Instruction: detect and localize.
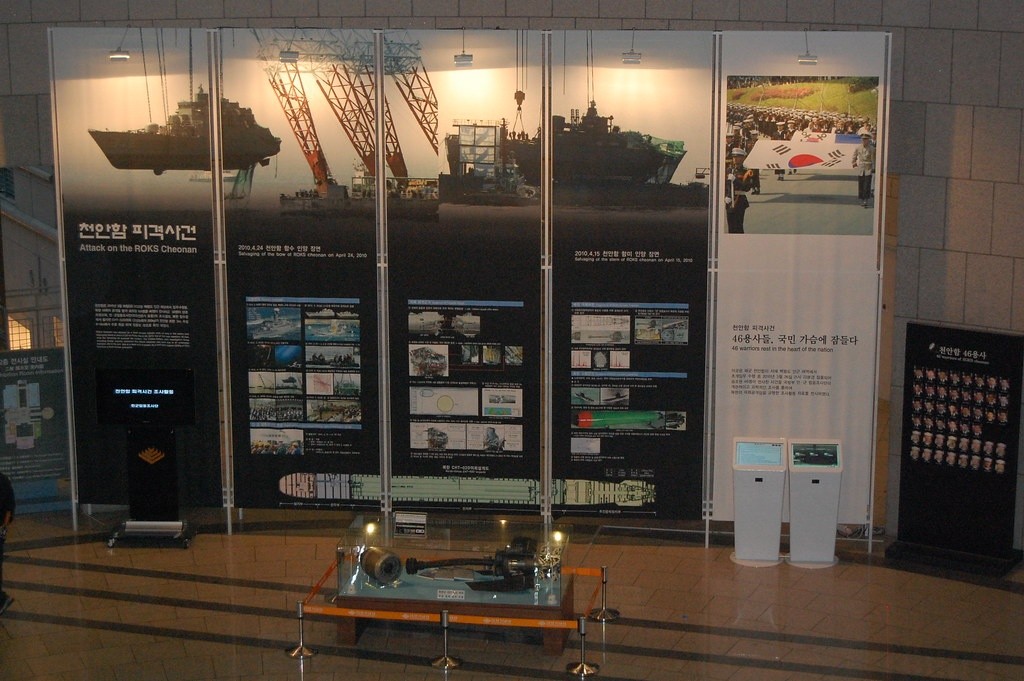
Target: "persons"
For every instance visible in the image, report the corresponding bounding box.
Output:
[724,99,876,236]
[310,351,355,368]
[251,439,304,456]
[315,403,362,424]
[908,365,1008,475]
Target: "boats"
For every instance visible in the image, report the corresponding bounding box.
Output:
[441,104,688,186]
[88,85,283,177]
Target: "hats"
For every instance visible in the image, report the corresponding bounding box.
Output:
[870,127,877,134]
[776,121,785,126]
[860,134,871,139]
[732,148,745,156]
[788,120,795,124]
[726,133,734,137]
[749,131,759,135]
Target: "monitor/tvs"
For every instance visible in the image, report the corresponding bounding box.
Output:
[735,442,781,466]
[791,444,839,467]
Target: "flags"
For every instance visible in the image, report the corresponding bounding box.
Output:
[740,138,865,174]
[789,130,864,147]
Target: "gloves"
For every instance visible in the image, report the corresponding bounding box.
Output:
[728,174,736,181]
[725,197,731,204]
[871,169,875,173]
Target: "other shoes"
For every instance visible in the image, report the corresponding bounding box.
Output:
[778,177,784,181]
[751,189,760,195]
